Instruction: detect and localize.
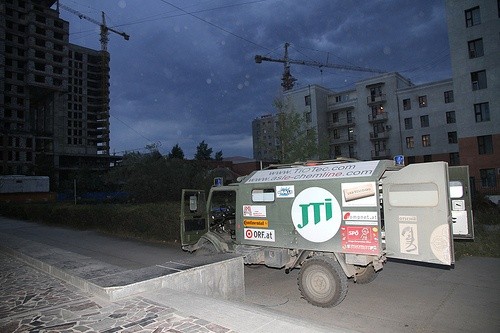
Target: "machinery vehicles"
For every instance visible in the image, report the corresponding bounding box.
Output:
[180,155,475,308]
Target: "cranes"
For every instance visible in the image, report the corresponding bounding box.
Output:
[56,2,130,51]
[254,41,387,90]
